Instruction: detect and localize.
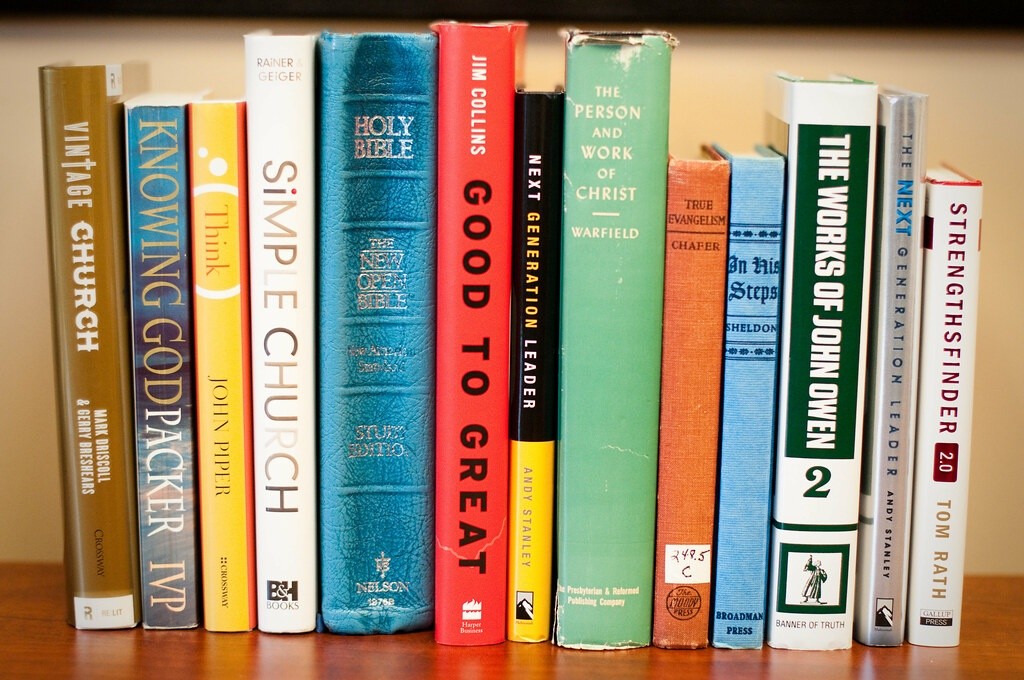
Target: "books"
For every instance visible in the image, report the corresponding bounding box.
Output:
[184,97,260,637]
[242,28,322,634]
[37,59,151,633]
[315,31,439,637]
[761,69,881,652]
[551,27,676,654]
[852,86,928,649]
[704,139,790,651]
[122,89,210,632]
[906,159,986,651]
[506,88,568,647]
[431,17,531,647]
[651,141,734,651]
